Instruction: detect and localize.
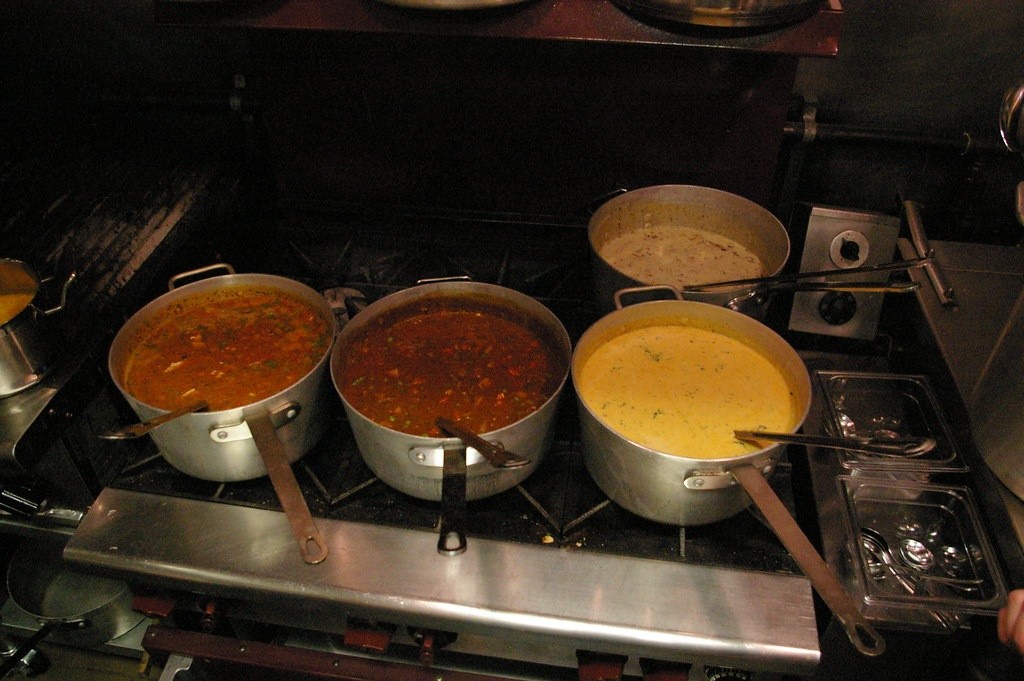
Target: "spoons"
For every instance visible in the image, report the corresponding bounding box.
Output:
[831,382,942,495]
[845,507,987,636]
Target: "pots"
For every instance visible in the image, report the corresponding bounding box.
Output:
[0,258,78,401]
[0,530,145,679]
[585,179,923,294]
[106,261,336,564]
[571,280,886,662]
[331,271,572,559]
